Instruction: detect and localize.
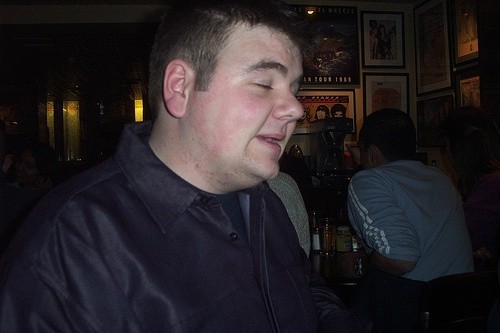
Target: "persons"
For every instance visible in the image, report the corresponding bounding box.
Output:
[14,143,59,192]
[346,107,475,285]
[389,26,397,60]
[0,119,47,257]
[0,0,365,333]
[378,24,389,60]
[369,27,379,59]
[369,20,377,29]
[442,106,500,271]
[266,172,310,258]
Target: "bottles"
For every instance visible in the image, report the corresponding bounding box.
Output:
[335,226,353,252]
[309,211,322,254]
[322,217,333,254]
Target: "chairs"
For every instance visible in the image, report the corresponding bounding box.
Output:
[288,144,347,229]
[417,271,500,333]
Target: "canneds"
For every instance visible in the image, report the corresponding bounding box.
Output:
[335,226,353,252]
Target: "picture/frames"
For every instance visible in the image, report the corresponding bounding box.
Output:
[412,0,454,98]
[454,65,481,108]
[416,92,454,149]
[449,0,479,68]
[292,88,357,136]
[359,10,407,70]
[285,4,360,84]
[362,71,411,121]
